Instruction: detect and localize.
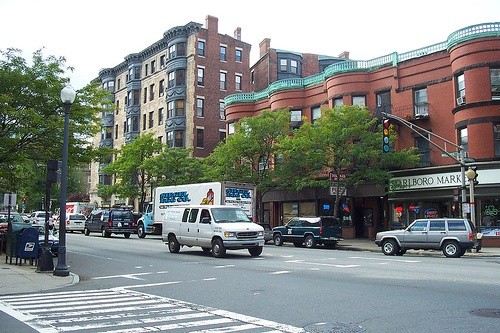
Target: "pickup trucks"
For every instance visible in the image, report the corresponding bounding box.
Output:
[162,205,266,259]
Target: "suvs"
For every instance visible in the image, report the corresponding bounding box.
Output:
[374,218,478,258]
[83,205,135,239]
[272,216,343,249]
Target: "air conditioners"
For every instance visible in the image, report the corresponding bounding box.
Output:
[457,96,466,106]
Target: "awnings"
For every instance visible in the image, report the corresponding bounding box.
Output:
[261,183,386,202]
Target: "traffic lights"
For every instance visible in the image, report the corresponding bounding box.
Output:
[469,165,478,187]
[382,116,391,154]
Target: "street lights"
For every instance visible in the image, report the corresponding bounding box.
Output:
[52,82,77,277]
[467,169,476,229]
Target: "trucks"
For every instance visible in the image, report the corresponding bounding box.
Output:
[136,180,256,238]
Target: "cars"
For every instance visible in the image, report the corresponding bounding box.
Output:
[257,221,275,245]
[0,210,53,244]
[52,213,87,234]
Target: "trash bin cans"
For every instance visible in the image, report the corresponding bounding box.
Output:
[6,221,39,258]
